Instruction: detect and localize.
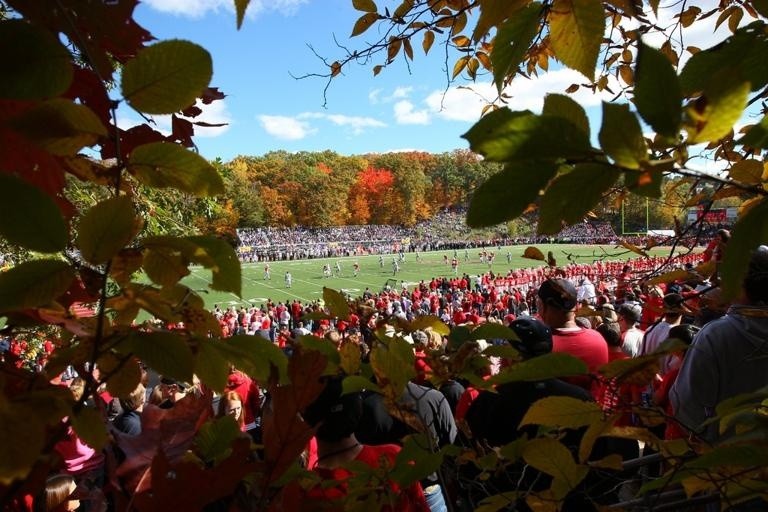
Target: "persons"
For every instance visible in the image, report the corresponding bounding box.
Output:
[0,220,768,512]
